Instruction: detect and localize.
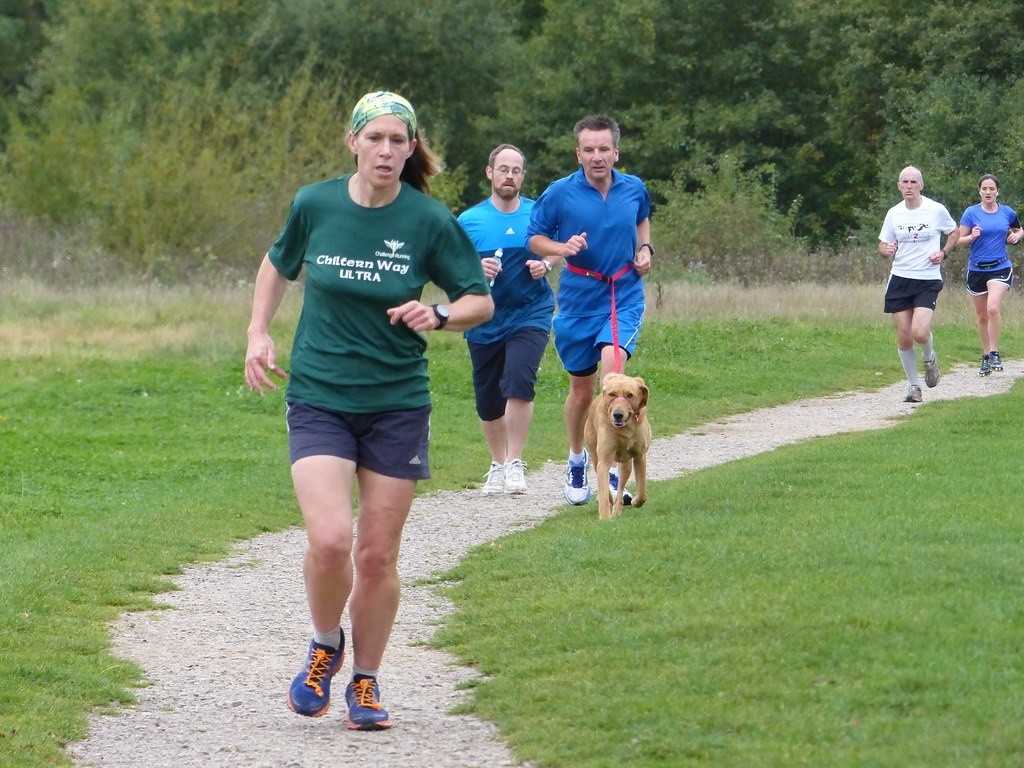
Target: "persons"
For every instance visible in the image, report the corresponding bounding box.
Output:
[241,89,496,732]
[523,112,654,508]
[878,164,961,403]
[953,173,1024,378]
[456,143,565,497]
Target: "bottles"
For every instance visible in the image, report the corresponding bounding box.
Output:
[486,248,503,287]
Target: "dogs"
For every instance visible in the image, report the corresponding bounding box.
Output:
[584,372,653,519]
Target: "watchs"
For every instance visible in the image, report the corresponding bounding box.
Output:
[940,249,949,260]
[638,243,655,256]
[541,259,553,274]
[429,304,449,331]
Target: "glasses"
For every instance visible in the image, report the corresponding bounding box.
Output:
[492,166,525,176]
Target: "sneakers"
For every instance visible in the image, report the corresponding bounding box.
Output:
[565,449,590,505]
[287,627,345,717]
[904,386,923,402]
[480,461,504,495]
[924,350,942,387]
[989,352,1003,371]
[345,674,392,729]
[503,458,527,493]
[979,355,992,377]
[597,472,633,505]
